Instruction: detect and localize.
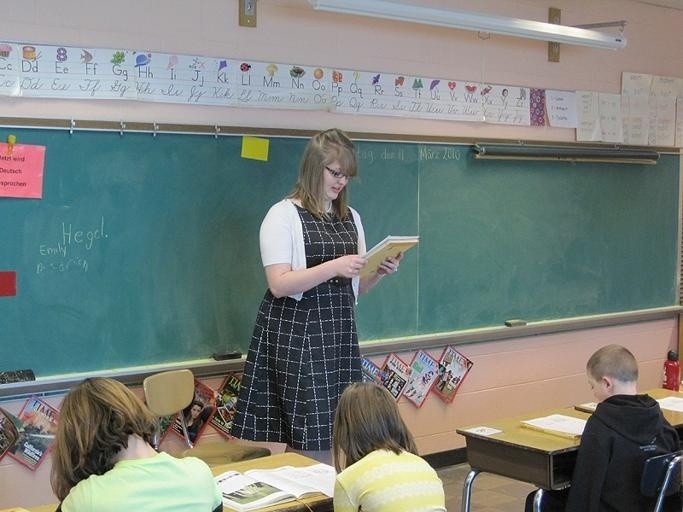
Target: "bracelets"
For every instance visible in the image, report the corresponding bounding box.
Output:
[375,271,385,278]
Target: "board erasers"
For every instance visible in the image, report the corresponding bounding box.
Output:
[213,350,242,361]
[505,320,527,327]
[0,369,35,384]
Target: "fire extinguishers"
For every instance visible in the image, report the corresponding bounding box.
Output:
[662,350,680,392]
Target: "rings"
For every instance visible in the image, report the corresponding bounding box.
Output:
[394,266,399,272]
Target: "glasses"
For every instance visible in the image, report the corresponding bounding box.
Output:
[325,166,353,179]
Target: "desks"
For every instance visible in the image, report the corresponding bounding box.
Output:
[456,385,682,512]
[208,451,343,512]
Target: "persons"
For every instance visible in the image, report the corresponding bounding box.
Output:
[231,129,403,463]
[523,344,683,512]
[49,376,223,512]
[333,383,447,512]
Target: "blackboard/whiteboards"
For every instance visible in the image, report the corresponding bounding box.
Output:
[0,115,683,398]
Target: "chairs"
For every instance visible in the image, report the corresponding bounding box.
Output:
[144,370,270,466]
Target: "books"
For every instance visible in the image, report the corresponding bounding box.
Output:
[574,402,599,413]
[520,413,589,440]
[464,426,502,436]
[213,463,339,512]
[655,396,683,412]
[353,232,420,269]
